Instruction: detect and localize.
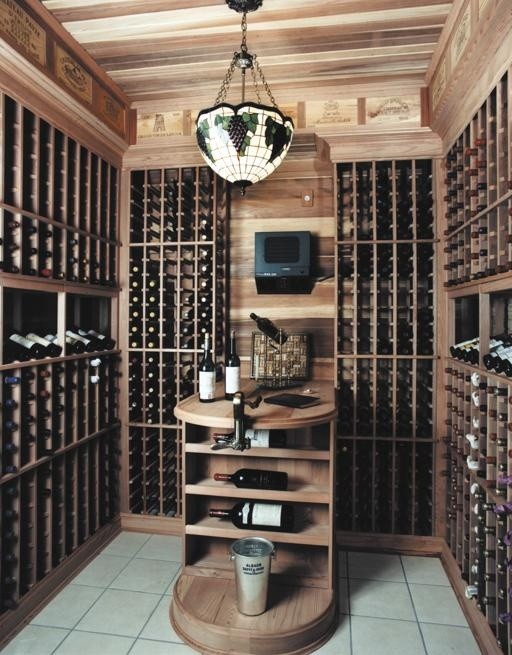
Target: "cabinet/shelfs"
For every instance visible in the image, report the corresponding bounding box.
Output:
[0,277,116,365]
[446,356,512,653]
[0,353,118,649]
[0,94,119,295]
[172,380,340,655]
[437,70,512,288]
[338,155,439,548]
[447,292,512,378]
[119,161,228,538]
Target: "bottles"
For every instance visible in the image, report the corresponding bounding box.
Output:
[3,430,123,610]
[208,500,304,532]
[4,322,116,360]
[212,428,287,448]
[198,332,217,402]
[440,452,512,648]
[3,355,121,471]
[128,262,157,348]
[443,137,512,235]
[449,332,512,377]
[181,249,223,349]
[4,219,116,287]
[250,312,288,345]
[441,366,512,512]
[224,330,241,401]
[130,352,193,424]
[214,468,289,490]
[198,219,212,241]
[442,226,511,286]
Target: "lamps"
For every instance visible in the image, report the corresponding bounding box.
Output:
[196,0,293,195]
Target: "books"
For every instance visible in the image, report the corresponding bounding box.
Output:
[264,393,322,409]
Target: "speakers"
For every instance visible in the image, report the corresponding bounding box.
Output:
[254,230,309,276]
